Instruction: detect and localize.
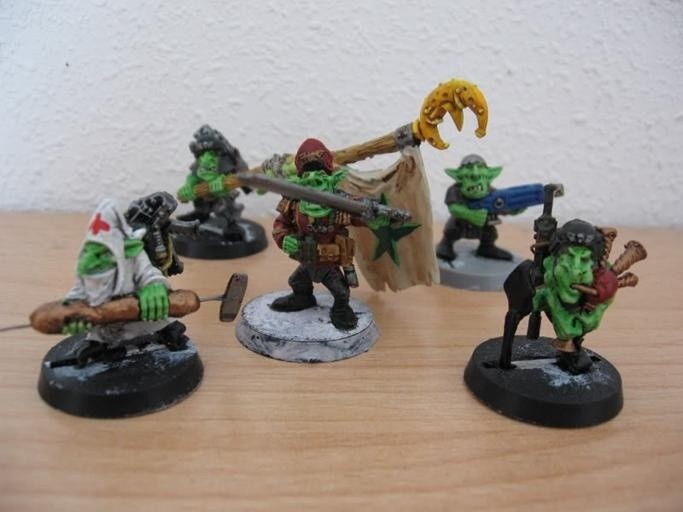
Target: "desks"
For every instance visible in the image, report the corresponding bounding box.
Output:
[1,214,680,510]
